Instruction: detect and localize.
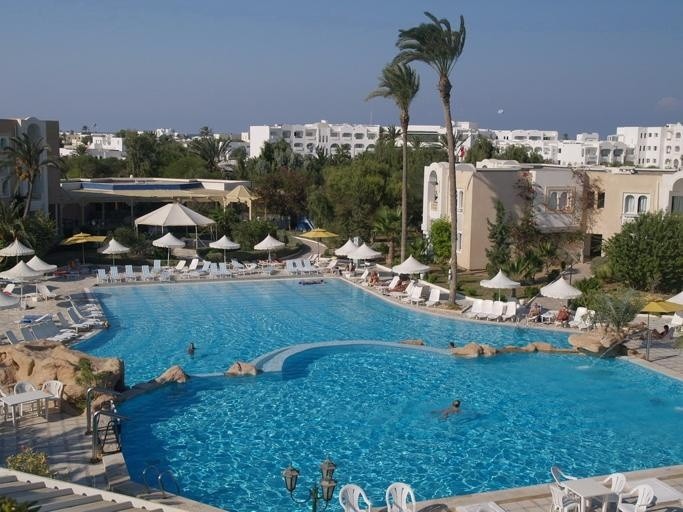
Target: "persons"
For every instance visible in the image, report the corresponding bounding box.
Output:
[652,325,668,338]
[345,262,354,271]
[557,305,569,322]
[369,271,378,285]
[299,280,323,285]
[528,303,538,318]
[385,276,410,292]
[449,342,456,348]
[105,320,108,327]
[189,343,194,353]
[441,400,460,419]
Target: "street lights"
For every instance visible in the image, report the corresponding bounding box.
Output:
[283,457,337,512]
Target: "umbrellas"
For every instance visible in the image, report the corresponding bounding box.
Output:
[540,276,583,305]
[639,299,683,361]
[64,232,99,264]
[299,227,339,262]
[152,232,185,265]
[480,268,520,301]
[392,254,430,278]
[254,233,285,263]
[335,237,382,269]
[0,237,57,308]
[209,235,241,263]
[97,237,130,266]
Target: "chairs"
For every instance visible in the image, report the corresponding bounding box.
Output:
[97,259,321,285]
[549,466,654,512]
[336,481,416,511]
[654,326,675,342]
[469,297,519,327]
[1,381,67,428]
[4,282,102,346]
[525,304,596,331]
[327,256,441,311]
